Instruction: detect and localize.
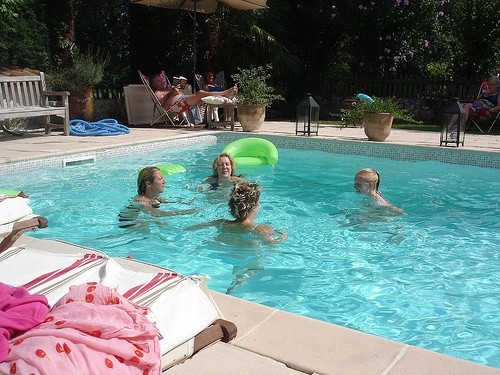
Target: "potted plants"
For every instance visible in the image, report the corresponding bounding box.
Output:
[230,64,282,132]
[340,95,419,142]
[52,49,107,120]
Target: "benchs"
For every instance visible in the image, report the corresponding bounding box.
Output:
[0,72,71,136]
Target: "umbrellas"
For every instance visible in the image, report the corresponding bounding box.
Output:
[132,0,270,94]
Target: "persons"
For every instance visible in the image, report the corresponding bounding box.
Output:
[150,72,238,122]
[119,152,286,244]
[354,169,386,204]
[447,77,500,138]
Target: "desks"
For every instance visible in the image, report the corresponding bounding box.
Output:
[206,101,236,130]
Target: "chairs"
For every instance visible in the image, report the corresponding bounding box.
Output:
[139,70,197,128]
[471,73,500,134]
[195,71,235,127]
